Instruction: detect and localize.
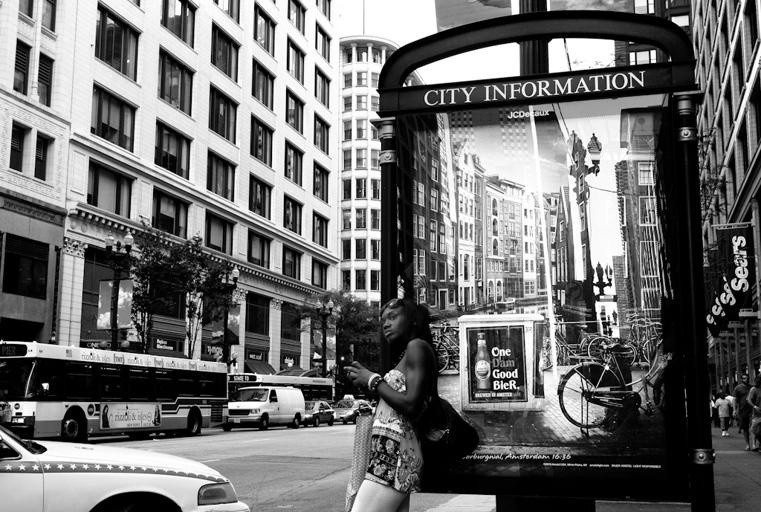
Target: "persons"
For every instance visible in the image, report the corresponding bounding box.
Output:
[709,372,761,452]
[150,405,160,425]
[345,297,441,512]
[101,404,110,428]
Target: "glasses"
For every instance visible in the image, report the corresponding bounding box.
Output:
[379,298,400,316]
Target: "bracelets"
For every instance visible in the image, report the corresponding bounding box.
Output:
[372,377,384,393]
[368,374,381,388]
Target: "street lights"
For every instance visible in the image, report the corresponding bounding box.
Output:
[606,311,617,336]
[591,259,611,337]
[106,229,135,351]
[316,297,334,377]
[221,263,241,362]
[565,129,602,332]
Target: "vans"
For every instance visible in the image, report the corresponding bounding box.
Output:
[222,384,307,432]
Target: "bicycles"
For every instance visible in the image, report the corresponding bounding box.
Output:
[543,312,668,439]
[430,320,459,375]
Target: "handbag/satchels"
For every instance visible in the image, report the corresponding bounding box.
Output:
[420,396,479,464]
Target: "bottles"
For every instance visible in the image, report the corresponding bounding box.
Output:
[470,333,494,392]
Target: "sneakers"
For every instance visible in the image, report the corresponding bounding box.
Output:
[722,430,729,437]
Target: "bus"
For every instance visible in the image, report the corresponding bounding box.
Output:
[0,340,228,441]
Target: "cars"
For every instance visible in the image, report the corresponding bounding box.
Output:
[305,399,335,427]
[0,420,250,511]
[333,394,372,425]
